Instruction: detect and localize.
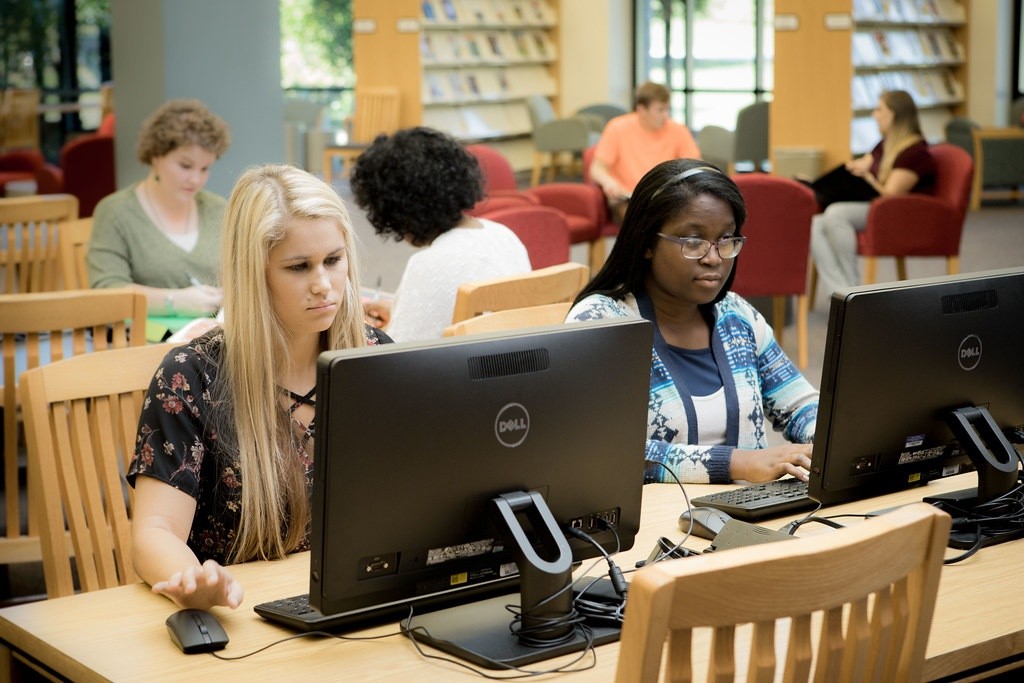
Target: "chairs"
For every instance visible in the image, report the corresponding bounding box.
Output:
[614,502,953,681]
[0,87,1024,596]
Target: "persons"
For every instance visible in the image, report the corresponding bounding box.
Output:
[125,165,395,612]
[811,90,931,305]
[563,159,822,485]
[588,81,704,225]
[349,127,533,344]
[87,98,231,323]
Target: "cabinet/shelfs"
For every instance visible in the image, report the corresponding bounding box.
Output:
[349,2,561,168]
[770,1,974,182]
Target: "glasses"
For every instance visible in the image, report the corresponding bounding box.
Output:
[659,233,747,259]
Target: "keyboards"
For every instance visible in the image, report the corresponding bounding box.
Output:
[254,563,583,637]
[690,475,809,521]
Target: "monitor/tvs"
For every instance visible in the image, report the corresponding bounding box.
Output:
[812,265,1024,550]
[309,316,654,667]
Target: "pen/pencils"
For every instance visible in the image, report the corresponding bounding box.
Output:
[368,310,384,322]
[185,272,200,285]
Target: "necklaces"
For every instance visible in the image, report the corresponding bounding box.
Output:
[142,179,194,247]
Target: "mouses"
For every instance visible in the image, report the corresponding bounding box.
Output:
[679,508,732,541]
[166,609,229,654]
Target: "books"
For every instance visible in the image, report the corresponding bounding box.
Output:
[418,0,554,100]
[852,0,967,154]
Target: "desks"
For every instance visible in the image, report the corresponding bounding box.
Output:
[1,453,1023,683]
